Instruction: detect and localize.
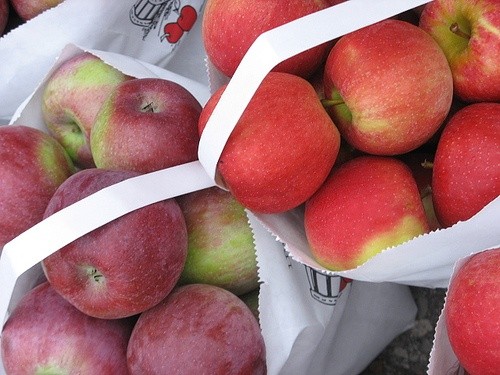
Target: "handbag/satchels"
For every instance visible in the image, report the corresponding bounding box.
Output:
[427,246,499,375]
[198,2,500,289]
[0,0,210,123]
[0,42,417,375]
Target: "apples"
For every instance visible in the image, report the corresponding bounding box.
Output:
[0,2,8,33]
[126,284,266,375]
[178,190,258,295]
[2,125,74,254]
[193,71,339,211]
[88,78,202,169]
[2,283,131,374]
[13,0,57,23]
[42,52,135,169]
[160,24,183,43]
[305,160,427,269]
[202,1,325,76]
[174,5,196,32]
[419,0,500,103]
[39,168,186,322]
[322,18,449,156]
[447,251,500,374]
[431,103,500,226]
